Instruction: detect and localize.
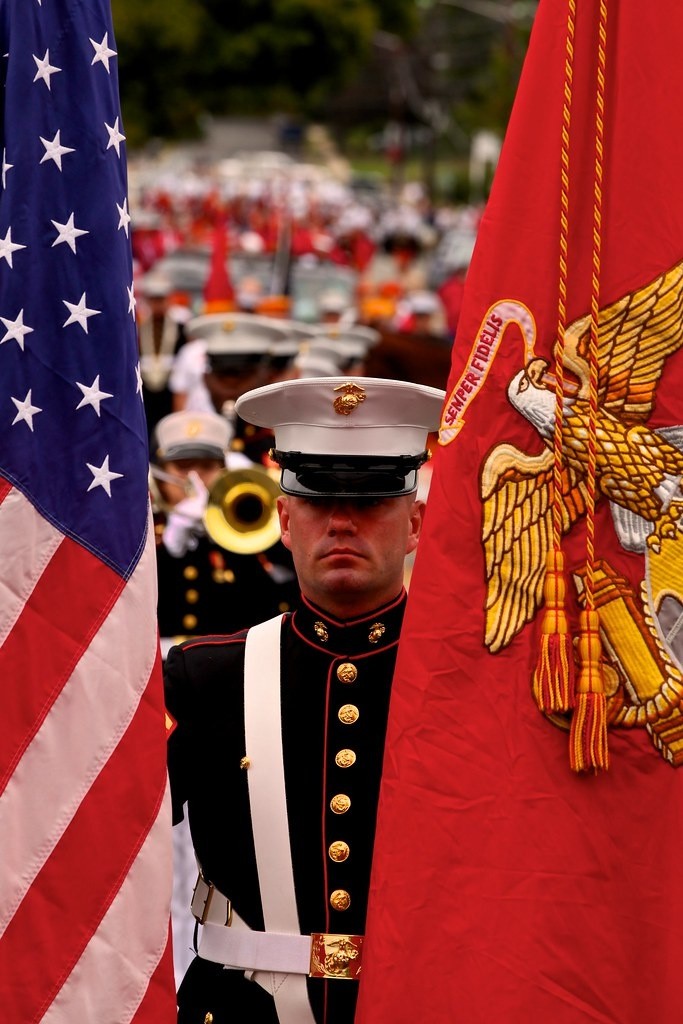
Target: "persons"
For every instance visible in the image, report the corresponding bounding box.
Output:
[116,136,487,1024]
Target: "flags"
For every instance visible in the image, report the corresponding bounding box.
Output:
[0,1,177,1024]
[352,0,683,1024]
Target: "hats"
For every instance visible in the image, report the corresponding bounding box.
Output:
[155,409,233,460]
[183,311,315,378]
[234,376,447,497]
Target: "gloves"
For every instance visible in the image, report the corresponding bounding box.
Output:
[161,470,210,558]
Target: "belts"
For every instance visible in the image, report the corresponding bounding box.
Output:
[198,923,365,980]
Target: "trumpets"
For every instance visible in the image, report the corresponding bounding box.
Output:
[202,469,287,556]
